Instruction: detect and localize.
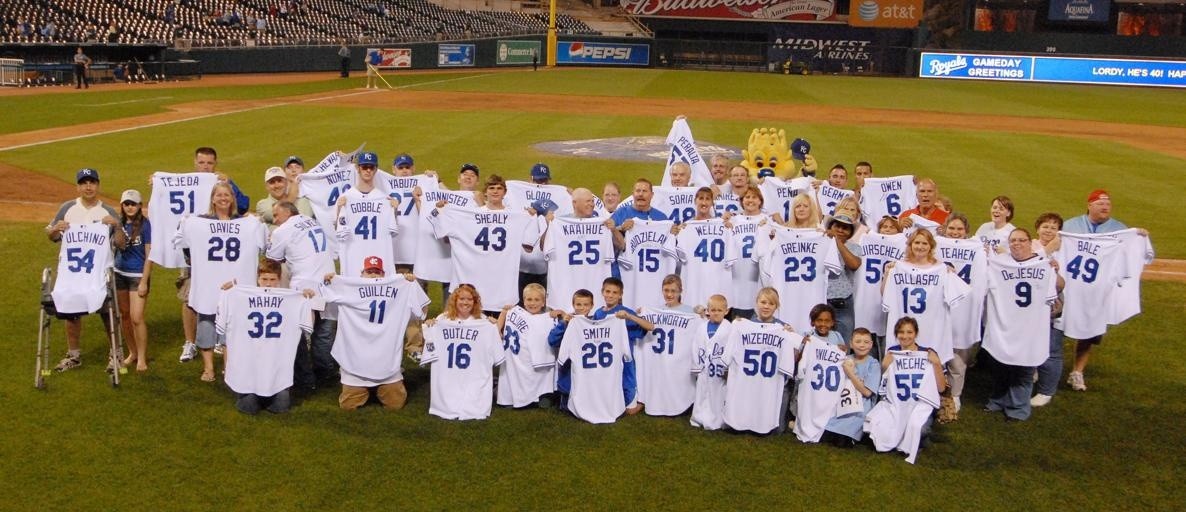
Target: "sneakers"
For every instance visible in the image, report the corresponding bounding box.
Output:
[54,340,225,384]
[1066,370,1086,392]
[1031,393,1052,408]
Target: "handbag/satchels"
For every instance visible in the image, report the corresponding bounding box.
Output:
[830,298,846,309]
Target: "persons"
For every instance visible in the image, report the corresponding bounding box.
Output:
[45,168,128,379]
[269,0,307,19]
[173,183,265,384]
[338,42,352,78]
[208,8,267,38]
[258,114,1063,453]
[365,47,384,89]
[148,147,250,362]
[113,60,151,85]
[109,187,152,374]
[1063,189,1148,393]
[532,49,537,71]
[216,259,314,416]
[73,47,91,90]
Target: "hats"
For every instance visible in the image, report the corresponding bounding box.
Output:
[531,199,557,216]
[361,257,384,271]
[825,210,860,232]
[792,138,810,159]
[1086,190,1109,214]
[77,168,98,184]
[532,163,551,182]
[265,151,479,180]
[120,190,141,204]
